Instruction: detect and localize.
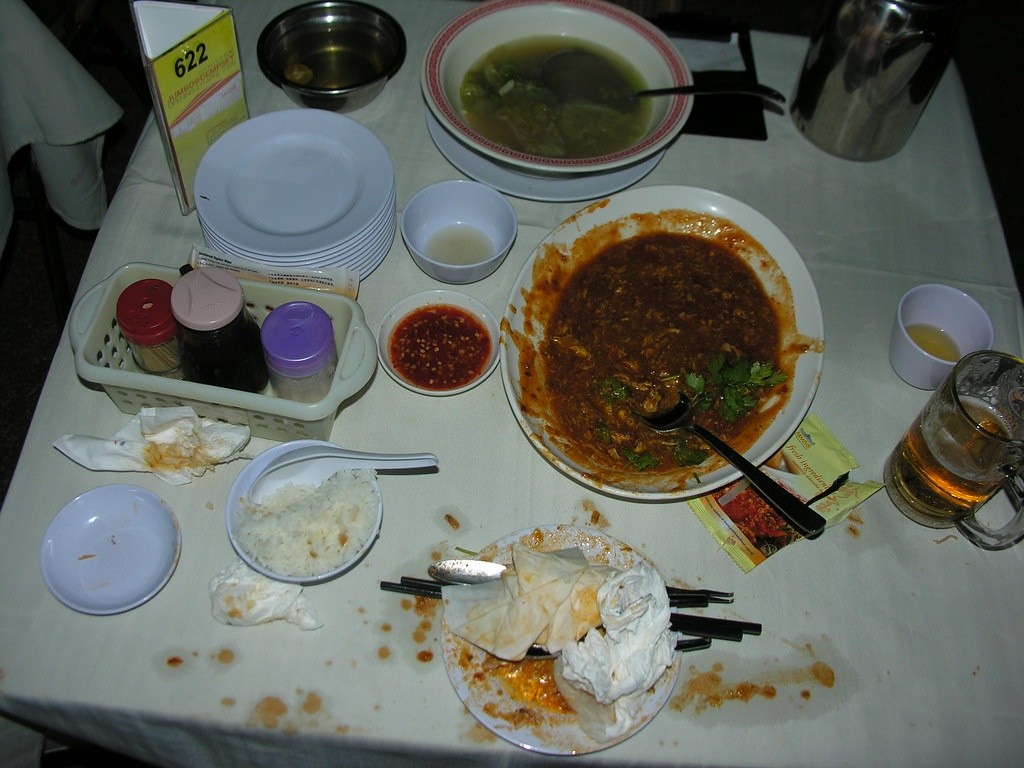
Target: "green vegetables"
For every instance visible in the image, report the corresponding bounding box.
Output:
[589,353,790,470]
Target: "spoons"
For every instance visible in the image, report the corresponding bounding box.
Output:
[247,445,439,507]
[627,389,827,539]
[542,48,786,107]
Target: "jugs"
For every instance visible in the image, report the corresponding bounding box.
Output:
[790,0,958,163]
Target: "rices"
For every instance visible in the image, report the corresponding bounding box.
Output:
[231,467,381,579]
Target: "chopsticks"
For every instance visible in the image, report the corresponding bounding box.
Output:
[378,577,762,642]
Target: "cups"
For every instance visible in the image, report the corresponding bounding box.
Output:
[889,283,995,390]
[883,350,1024,550]
[170,263,269,393]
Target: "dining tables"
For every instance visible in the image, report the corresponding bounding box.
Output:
[0,0,1024,768]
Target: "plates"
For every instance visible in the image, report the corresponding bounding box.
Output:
[192,109,397,282]
[440,524,683,757]
[426,103,670,203]
[375,288,501,398]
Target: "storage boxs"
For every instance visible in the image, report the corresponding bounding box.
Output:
[67,261,379,444]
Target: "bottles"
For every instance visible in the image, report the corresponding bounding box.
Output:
[259,301,338,403]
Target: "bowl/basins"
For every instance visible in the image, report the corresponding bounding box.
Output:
[498,185,825,502]
[421,0,695,175]
[256,1,407,113]
[225,439,383,585]
[400,179,517,284]
[40,484,182,616]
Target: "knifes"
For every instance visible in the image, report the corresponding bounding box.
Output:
[427,559,710,606]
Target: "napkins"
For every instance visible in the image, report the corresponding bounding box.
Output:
[52,406,256,486]
[441,547,682,742]
[209,560,322,630]
